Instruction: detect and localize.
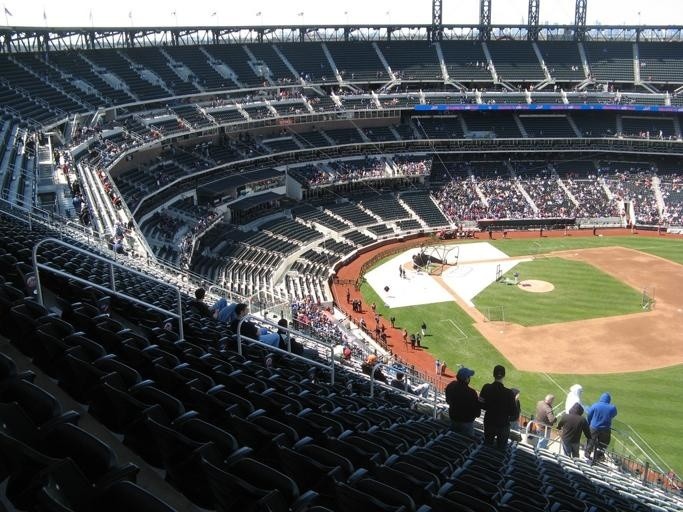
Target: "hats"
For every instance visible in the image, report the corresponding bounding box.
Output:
[456,368,473,380]
[493,366,504,378]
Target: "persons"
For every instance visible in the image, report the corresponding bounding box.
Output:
[16,135,24,146]
[558,401,592,458]
[228,302,281,351]
[177,241,194,276]
[108,237,114,251]
[52,53,681,240]
[190,287,236,325]
[274,318,320,361]
[291,260,448,402]
[443,365,481,437]
[114,238,128,257]
[512,271,519,282]
[531,393,557,449]
[476,364,519,451]
[562,383,589,417]
[584,393,618,462]
